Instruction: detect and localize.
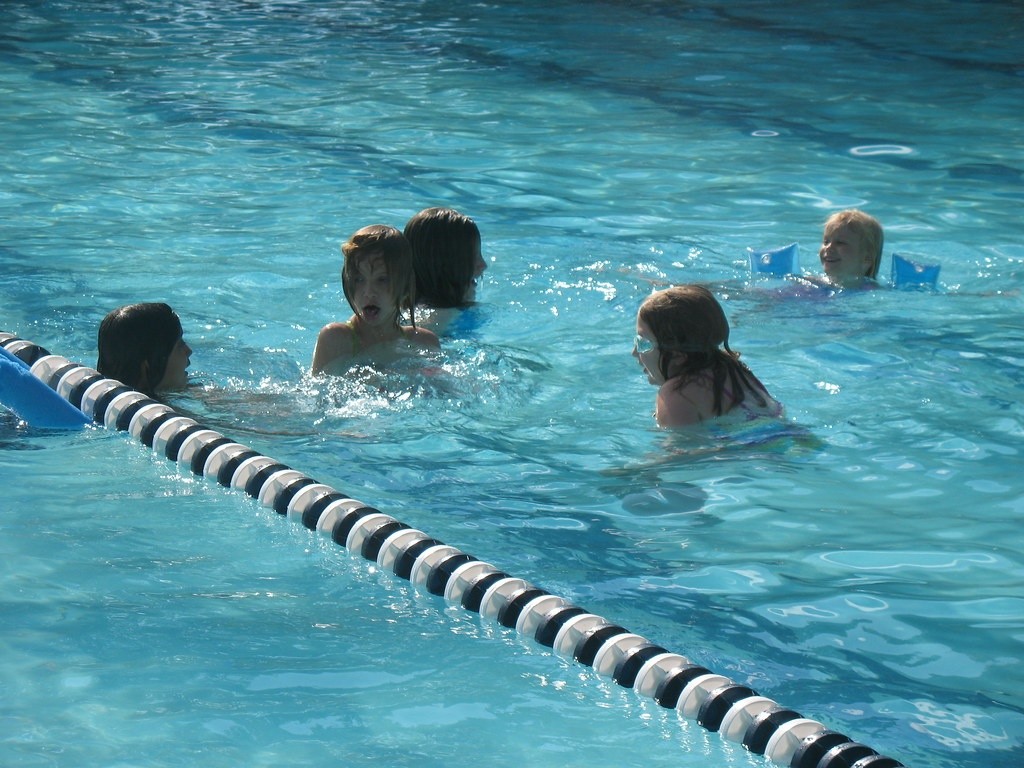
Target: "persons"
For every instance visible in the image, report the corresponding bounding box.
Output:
[97,303,192,392]
[820,209,884,284]
[311,224,442,376]
[404,207,487,306]
[631,285,781,429]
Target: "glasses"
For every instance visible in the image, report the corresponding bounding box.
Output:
[634,334,721,356]
